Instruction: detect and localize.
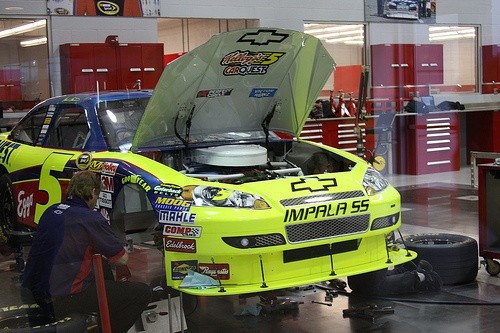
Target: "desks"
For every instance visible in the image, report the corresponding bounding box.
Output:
[392,102,500,173]
[305,115,380,123]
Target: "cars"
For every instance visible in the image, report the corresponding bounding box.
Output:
[0,27,418,317]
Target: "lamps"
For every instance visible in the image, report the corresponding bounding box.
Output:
[20,37,48,47]
[0,18,47,39]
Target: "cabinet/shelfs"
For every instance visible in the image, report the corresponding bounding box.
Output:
[60,42,164,96]
[0,69,24,103]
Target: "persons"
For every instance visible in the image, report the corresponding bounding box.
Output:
[348,260,442,297]
[23,171,152,333]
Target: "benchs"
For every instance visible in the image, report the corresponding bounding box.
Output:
[19,125,88,148]
